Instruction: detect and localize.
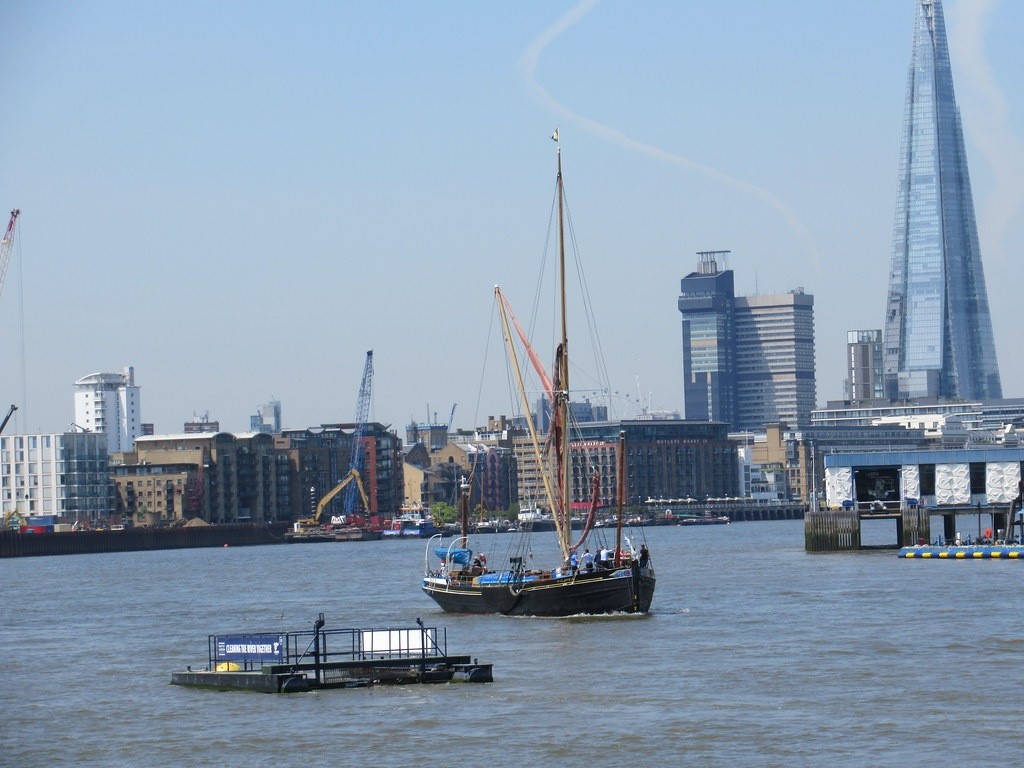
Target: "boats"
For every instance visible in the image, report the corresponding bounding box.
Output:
[167,610,495,694]
[381,496,730,539]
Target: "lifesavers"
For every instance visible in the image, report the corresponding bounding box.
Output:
[985,528,992,538]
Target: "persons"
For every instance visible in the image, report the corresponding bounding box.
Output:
[469,553,488,576]
[570,546,618,574]
[620,544,648,569]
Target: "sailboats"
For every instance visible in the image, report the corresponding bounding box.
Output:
[417,126,659,616]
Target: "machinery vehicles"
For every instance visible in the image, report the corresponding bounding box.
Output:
[291,468,373,532]
[330,347,375,526]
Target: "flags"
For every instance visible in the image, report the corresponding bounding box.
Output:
[552,127,559,142]
